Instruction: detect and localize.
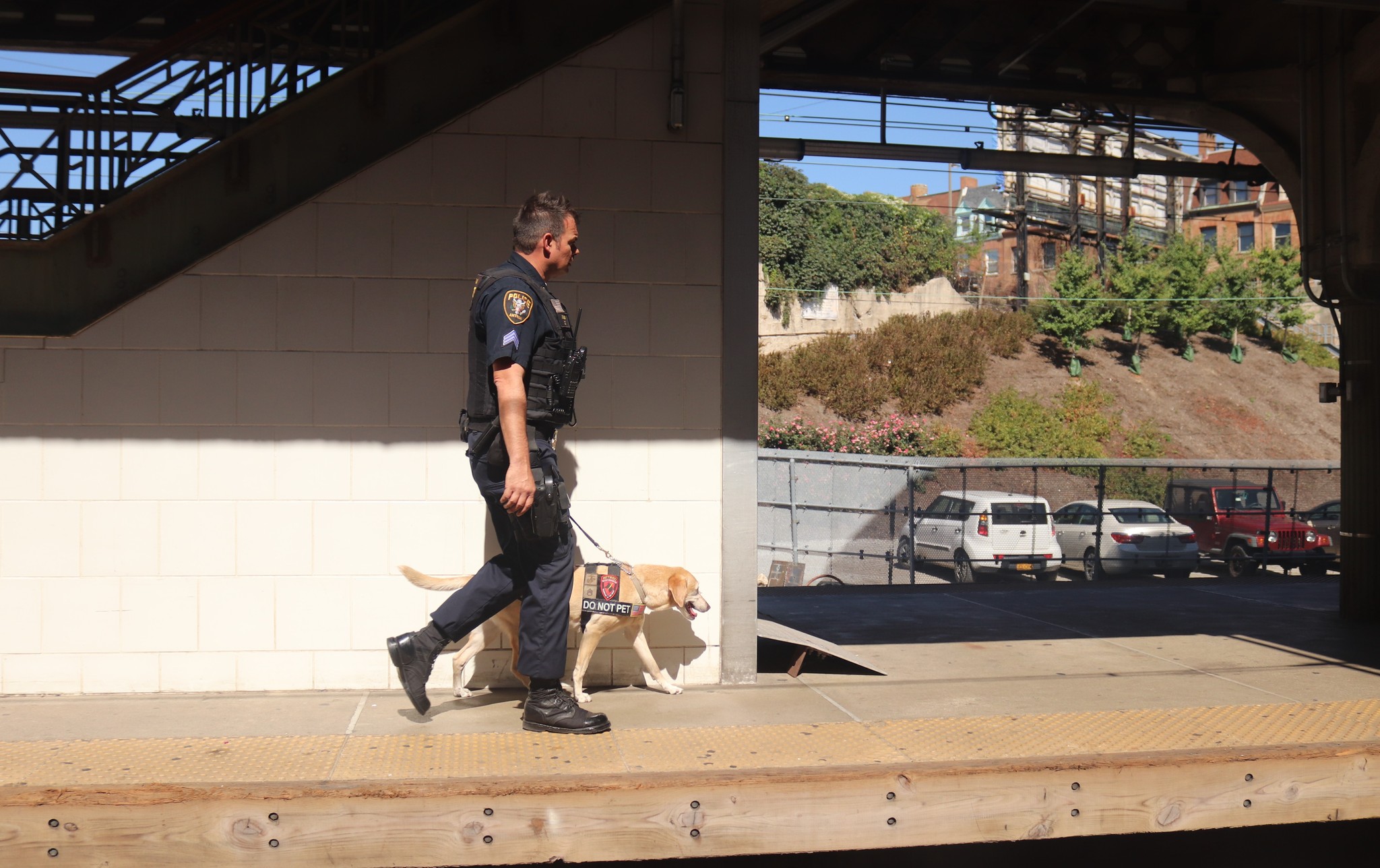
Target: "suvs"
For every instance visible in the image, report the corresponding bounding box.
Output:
[1166,479,1334,582]
[896,489,1062,587]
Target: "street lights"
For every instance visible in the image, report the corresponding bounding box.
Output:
[947,162,961,221]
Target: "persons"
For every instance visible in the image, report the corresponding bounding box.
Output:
[387,191,611,736]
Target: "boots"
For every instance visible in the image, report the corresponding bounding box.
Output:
[522,678,611,734]
[387,620,451,716]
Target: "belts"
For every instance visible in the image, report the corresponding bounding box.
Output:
[466,421,558,451]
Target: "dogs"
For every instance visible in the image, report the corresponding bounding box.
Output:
[396,560,711,702]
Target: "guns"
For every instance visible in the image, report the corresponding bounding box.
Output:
[530,482,547,500]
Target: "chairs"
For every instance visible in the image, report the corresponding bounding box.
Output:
[1143,513,1161,523]
[960,504,1034,522]
[1195,494,1258,509]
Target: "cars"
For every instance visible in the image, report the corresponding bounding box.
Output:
[1051,499,1198,585]
[1296,499,1341,561]
